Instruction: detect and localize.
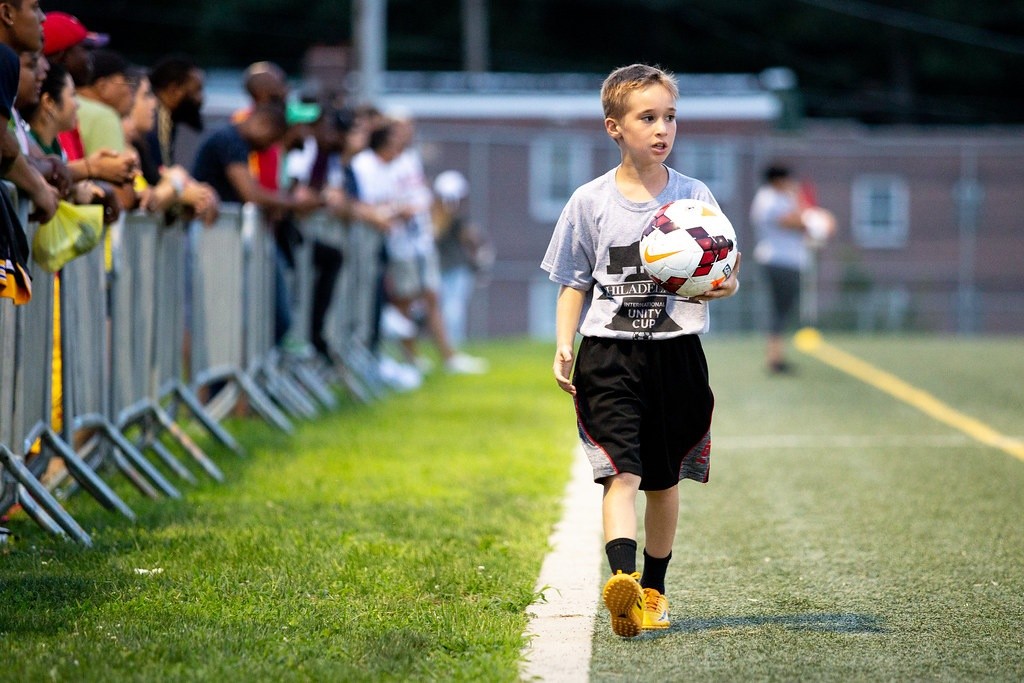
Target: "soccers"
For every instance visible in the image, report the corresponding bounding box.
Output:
[641,199,738,296]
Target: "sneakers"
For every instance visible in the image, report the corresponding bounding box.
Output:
[641,588,671,629]
[603,570,645,638]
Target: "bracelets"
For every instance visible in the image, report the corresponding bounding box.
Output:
[84,157,93,178]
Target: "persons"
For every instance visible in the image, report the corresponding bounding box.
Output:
[749,161,838,374]
[0,0,490,544]
[539,64,742,638]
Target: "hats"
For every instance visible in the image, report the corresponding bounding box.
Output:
[41,12,110,55]
